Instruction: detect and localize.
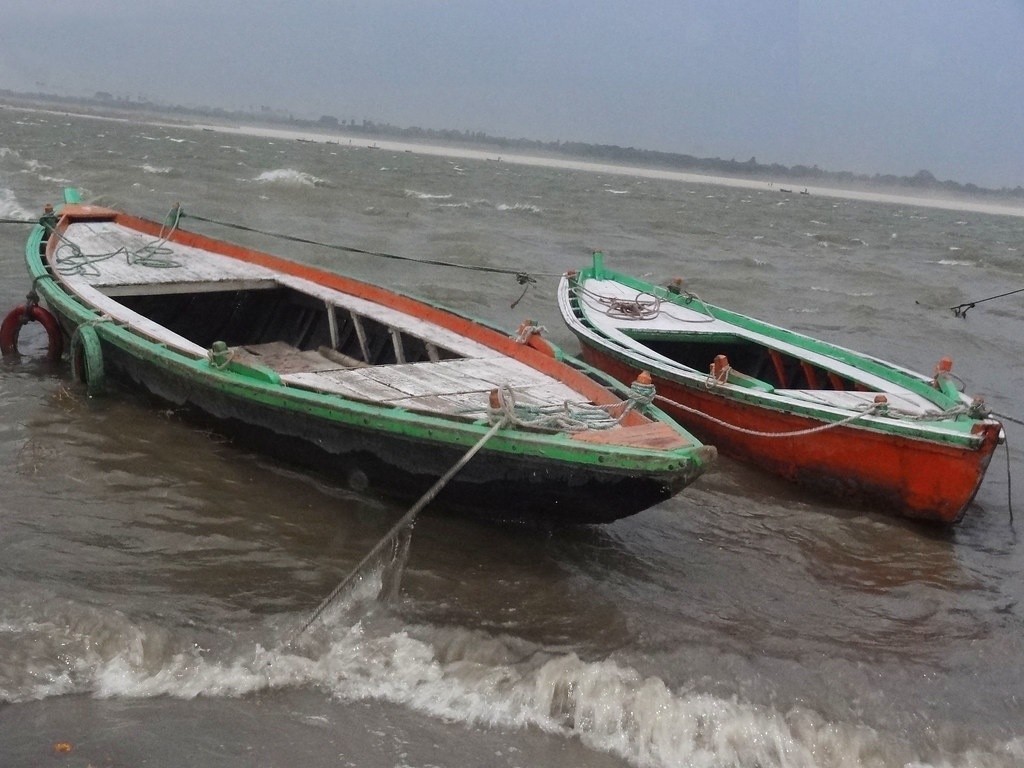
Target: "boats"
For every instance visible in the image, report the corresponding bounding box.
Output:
[555,250,1006,530]
[24,188,720,530]
